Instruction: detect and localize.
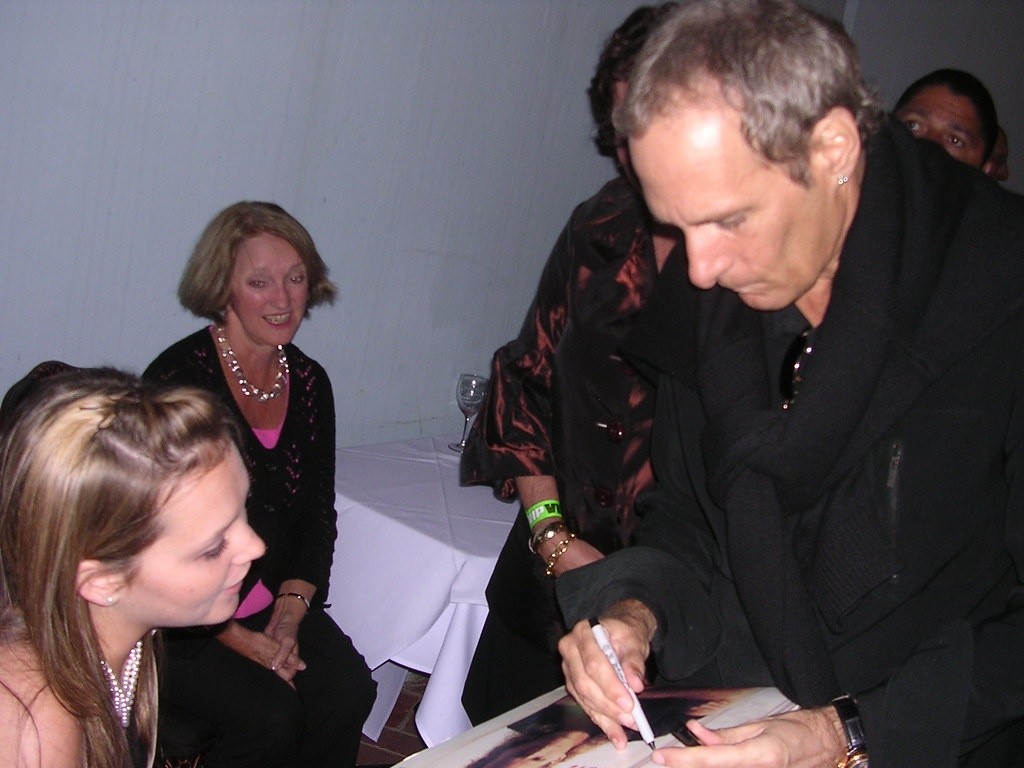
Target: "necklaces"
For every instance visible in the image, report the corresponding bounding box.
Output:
[214,322,294,399]
[98,640,146,731]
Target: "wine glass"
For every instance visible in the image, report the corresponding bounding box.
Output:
[448,373,489,453]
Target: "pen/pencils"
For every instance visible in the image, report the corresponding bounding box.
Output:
[587,616,656,751]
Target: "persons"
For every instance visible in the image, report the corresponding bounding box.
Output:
[0,359,265,768]
[473,691,668,768]
[890,68,996,168]
[984,124,1008,184]
[462,7,705,728]
[555,1,1024,768]
[139,202,374,768]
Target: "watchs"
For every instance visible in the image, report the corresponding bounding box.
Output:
[830,694,869,768]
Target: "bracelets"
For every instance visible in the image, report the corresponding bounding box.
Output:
[527,520,565,554]
[524,498,564,532]
[543,532,577,576]
[274,592,310,610]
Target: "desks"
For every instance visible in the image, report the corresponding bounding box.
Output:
[326,435,521,750]
